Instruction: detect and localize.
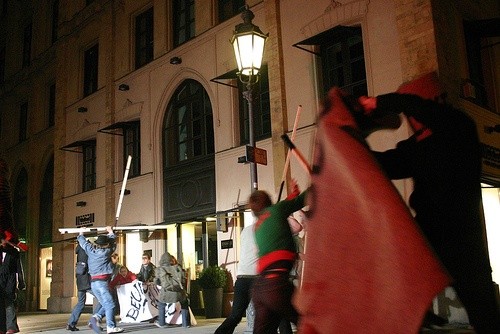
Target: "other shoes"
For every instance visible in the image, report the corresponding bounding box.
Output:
[65,324,79,331]
[5,327,20,334]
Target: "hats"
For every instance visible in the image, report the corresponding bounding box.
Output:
[93,235,109,245]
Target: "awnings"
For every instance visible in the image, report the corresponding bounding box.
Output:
[98,122,140,136]
[59,141,95,154]
[211,68,239,88]
[292,24,361,58]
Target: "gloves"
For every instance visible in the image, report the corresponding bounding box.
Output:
[339,93,415,131]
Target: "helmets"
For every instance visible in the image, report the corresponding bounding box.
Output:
[395,71,456,102]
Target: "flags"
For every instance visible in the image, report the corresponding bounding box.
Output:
[300,87,474,334]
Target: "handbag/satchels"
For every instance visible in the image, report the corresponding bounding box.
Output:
[75,261,86,276]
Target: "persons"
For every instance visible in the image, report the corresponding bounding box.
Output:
[109,253,193,328]
[0,227,20,334]
[358,71,500,334]
[214,179,308,334]
[66,226,123,334]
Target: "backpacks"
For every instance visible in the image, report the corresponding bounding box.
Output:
[159,266,181,293]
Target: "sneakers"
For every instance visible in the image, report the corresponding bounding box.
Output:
[87,312,104,333]
[106,325,125,333]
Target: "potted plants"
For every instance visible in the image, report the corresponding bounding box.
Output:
[195,264,229,319]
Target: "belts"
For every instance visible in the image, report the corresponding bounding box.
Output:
[237,275,255,281]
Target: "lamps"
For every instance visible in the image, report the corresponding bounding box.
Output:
[237,156,249,164]
[119,188,130,196]
[76,201,87,207]
[118,84,130,91]
[169,56,181,65]
[78,106,88,113]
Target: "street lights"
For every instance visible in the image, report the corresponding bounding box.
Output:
[227,4,271,193]
